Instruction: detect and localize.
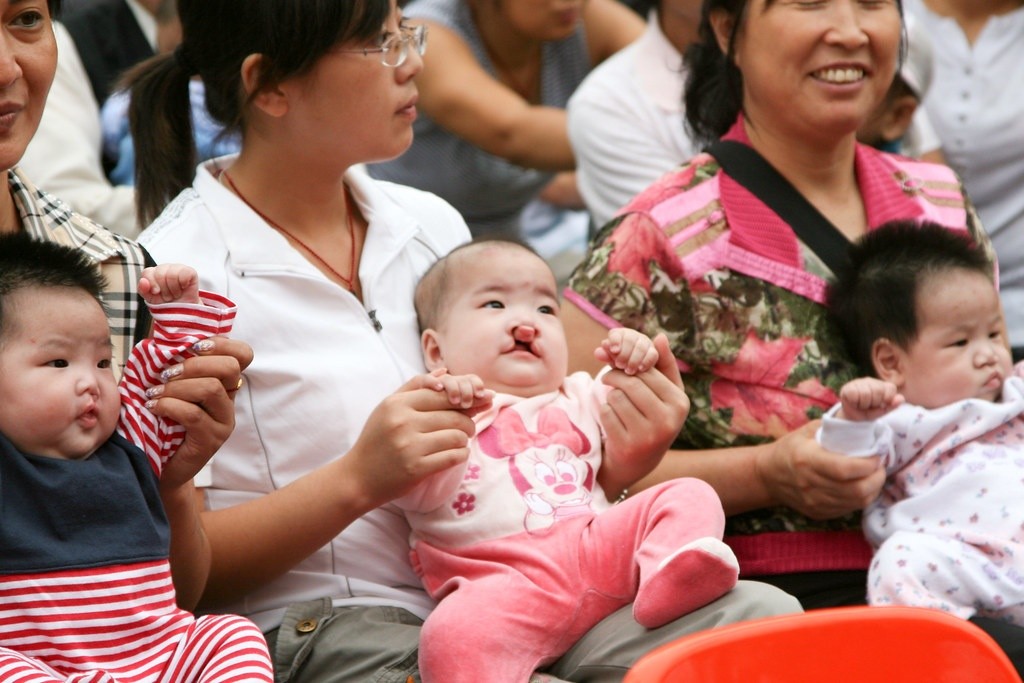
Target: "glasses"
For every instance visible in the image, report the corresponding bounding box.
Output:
[322,24,428,68]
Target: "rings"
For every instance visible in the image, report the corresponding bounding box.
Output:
[227,377,243,392]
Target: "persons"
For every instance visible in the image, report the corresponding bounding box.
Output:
[0,0,1024,683]
[0,232,276,683]
[385,239,740,683]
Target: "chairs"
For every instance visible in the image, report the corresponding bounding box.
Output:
[621,605,1021,683]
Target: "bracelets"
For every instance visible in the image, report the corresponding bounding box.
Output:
[613,489,628,504]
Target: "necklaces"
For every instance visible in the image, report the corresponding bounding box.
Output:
[472,6,525,95]
[224,172,358,296]
